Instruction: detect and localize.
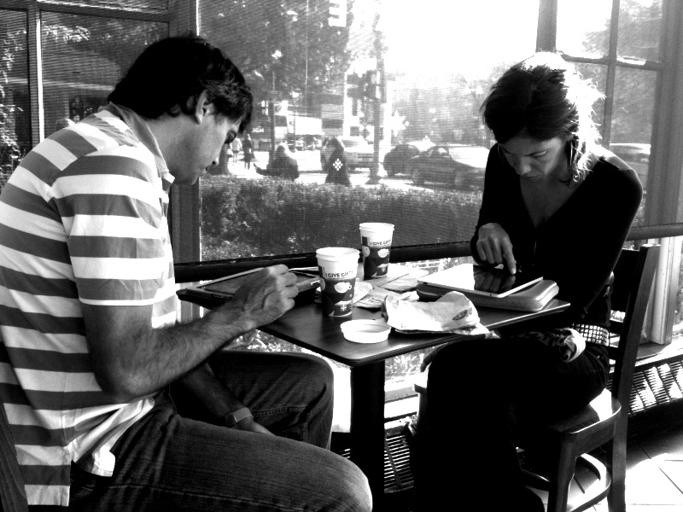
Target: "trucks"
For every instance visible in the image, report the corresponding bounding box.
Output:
[287,139,303,151]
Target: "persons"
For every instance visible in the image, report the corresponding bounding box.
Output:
[242,133,253,168]
[412,63,640,512]
[0,36,373,512]
[232,136,241,162]
[320,137,352,186]
[254,146,298,182]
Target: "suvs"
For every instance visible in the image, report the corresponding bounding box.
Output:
[315,134,373,172]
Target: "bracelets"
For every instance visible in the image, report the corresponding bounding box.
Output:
[224,407,252,427]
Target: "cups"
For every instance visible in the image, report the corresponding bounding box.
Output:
[357,222,398,278]
[314,245,360,318]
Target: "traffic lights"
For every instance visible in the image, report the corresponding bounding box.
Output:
[365,85,381,99]
[365,69,381,84]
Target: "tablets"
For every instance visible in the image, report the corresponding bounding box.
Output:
[187,266,321,303]
[417,263,544,301]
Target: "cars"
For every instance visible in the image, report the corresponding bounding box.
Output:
[609,139,651,194]
[400,141,490,191]
[376,139,441,179]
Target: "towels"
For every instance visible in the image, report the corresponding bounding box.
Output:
[381,291,496,339]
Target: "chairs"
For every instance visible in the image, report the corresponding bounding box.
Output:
[400,242,662,512]
